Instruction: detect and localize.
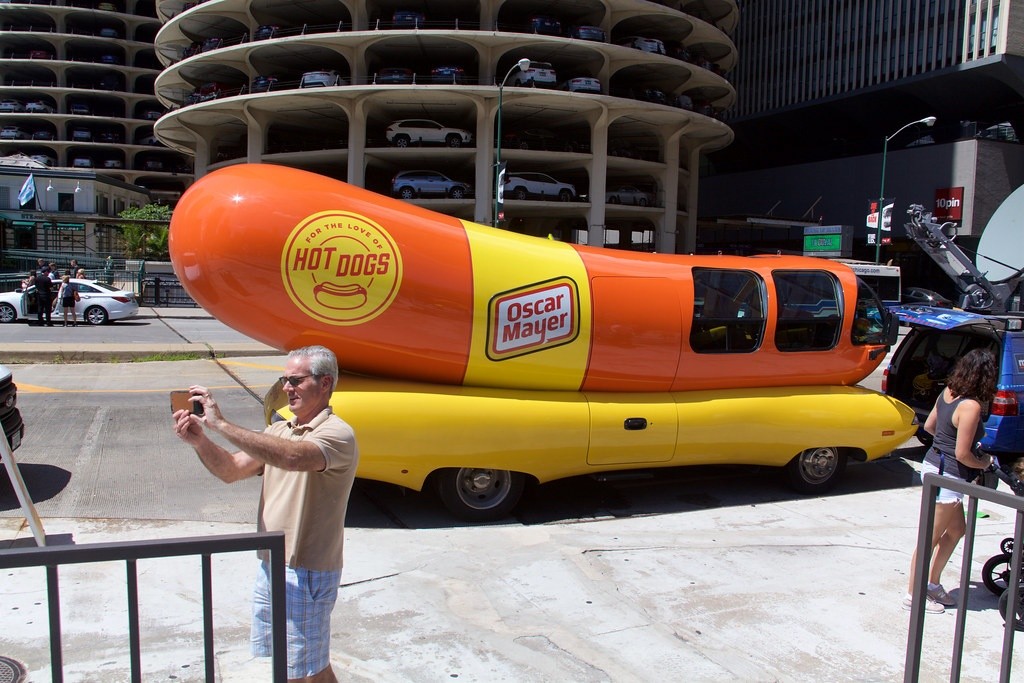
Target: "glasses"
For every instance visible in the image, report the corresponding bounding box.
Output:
[279,373,317,386]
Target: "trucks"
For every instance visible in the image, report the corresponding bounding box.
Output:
[165,166,923,524]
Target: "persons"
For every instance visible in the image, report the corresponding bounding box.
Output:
[76,268,87,279]
[65,270,71,277]
[41,266,56,309]
[37,258,49,270]
[902,348,995,614]
[58,275,79,328]
[22,269,38,305]
[104,255,114,285]
[1013,457,1024,484]
[51,283,61,312]
[49,263,60,279]
[172,346,358,683]
[70,260,79,278]
[36,266,55,327]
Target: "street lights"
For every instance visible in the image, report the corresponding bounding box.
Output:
[868,113,938,307]
[491,58,531,226]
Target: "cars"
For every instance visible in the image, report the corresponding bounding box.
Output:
[1,276,138,327]
[173,0,724,122]
[880,204,1023,488]
[0,0,180,191]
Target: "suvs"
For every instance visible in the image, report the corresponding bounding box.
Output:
[386,117,472,146]
[390,168,472,200]
[504,167,576,203]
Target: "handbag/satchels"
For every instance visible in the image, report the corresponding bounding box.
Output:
[74,291,80,302]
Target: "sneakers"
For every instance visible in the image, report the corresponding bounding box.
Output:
[901,584,955,614]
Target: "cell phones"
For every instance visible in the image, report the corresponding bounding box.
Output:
[170,391,205,417]
